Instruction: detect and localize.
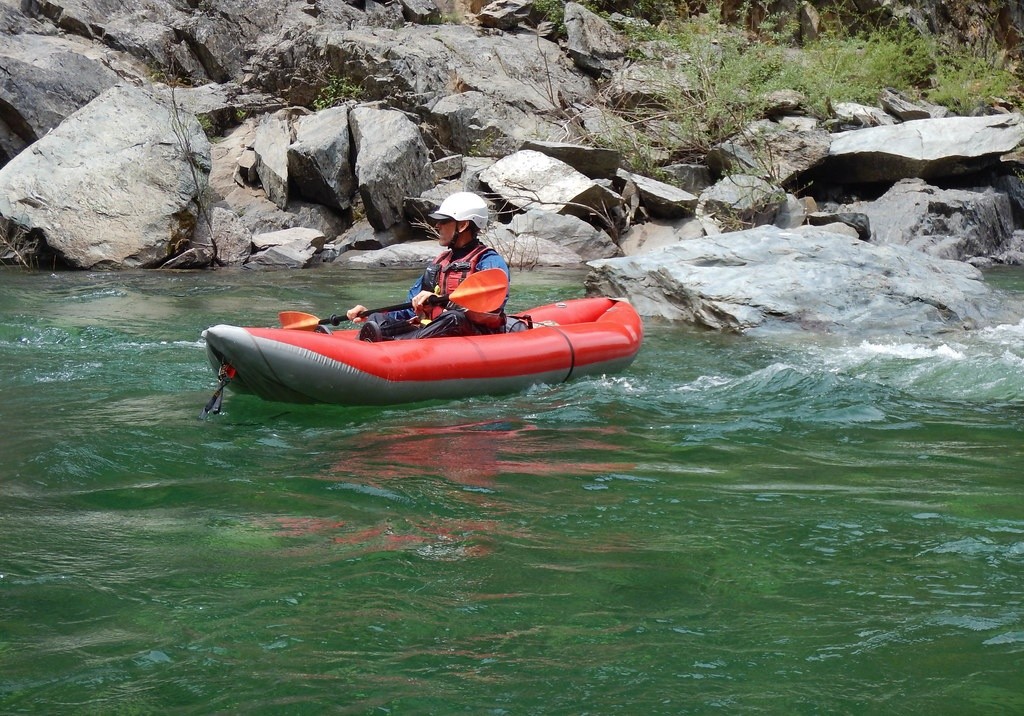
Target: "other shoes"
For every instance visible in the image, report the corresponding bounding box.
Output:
[360,321,382,343]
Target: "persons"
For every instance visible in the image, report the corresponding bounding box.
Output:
[347,192,510,342]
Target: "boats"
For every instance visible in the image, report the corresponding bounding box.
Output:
[200,296,645,407]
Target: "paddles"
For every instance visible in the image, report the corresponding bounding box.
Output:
[279,267,508,333]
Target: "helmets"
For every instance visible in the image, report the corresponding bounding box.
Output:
[435,192,487,229]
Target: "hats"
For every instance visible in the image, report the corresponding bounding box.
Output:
[428,213,451,220]
